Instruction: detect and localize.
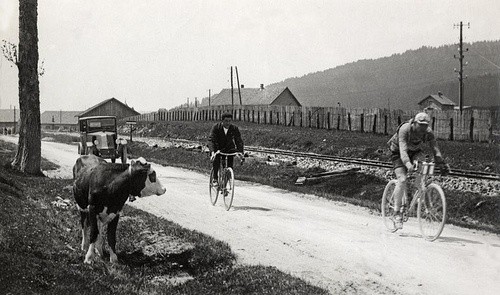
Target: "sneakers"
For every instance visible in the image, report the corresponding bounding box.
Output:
[392,213,403,229]
[211,178,218,187]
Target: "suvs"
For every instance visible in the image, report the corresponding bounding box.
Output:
[78,116,127,163]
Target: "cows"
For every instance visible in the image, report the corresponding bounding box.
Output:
[72,154,166,265]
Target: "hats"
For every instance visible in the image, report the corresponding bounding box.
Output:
[414,112,431,124]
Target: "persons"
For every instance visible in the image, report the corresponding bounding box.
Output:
[385,112,444,228]
[207,112,245,196]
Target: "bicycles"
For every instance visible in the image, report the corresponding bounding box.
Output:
[381,160,447,242]
[210,150,245,211]
